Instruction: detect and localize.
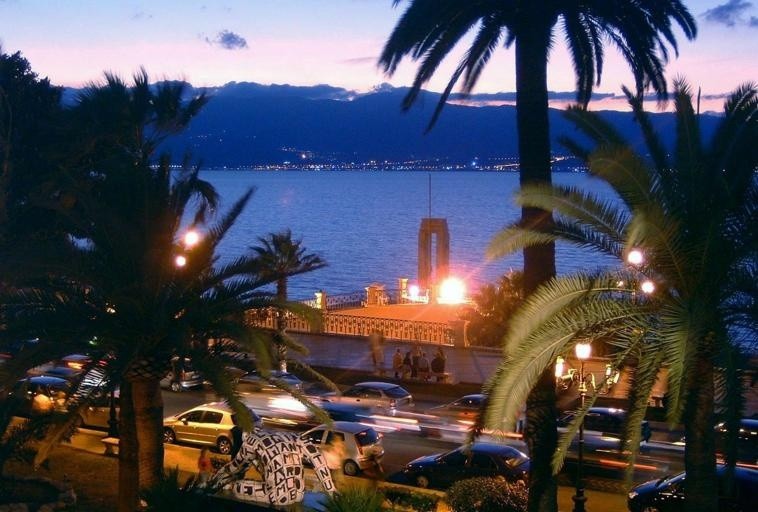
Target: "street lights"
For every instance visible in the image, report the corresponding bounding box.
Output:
[555,340,620,511]
[615,248,656,312]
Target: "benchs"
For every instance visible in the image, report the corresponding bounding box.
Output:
[374,365,454,385]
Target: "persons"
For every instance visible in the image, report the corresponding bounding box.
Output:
[402,351,412,379]
[55,390,73,445]
[412,343,423,378]
[391,348,403,379]
[437,346,447,379]
[32,388,53,415]
[416,351,431,379]
[430,351,441,382]
[198,446,216,472]
[369,328,381,364]
[228,425,243,461]
